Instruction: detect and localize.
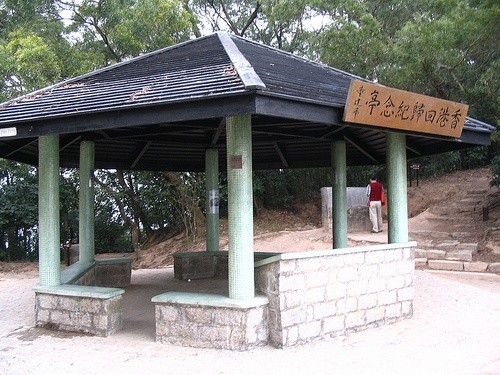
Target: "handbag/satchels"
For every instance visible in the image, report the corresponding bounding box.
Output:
[381,187,385,207]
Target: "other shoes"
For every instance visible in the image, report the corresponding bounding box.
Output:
[370,230,382,233]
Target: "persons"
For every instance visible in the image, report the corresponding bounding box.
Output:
[366,175,385,234]
